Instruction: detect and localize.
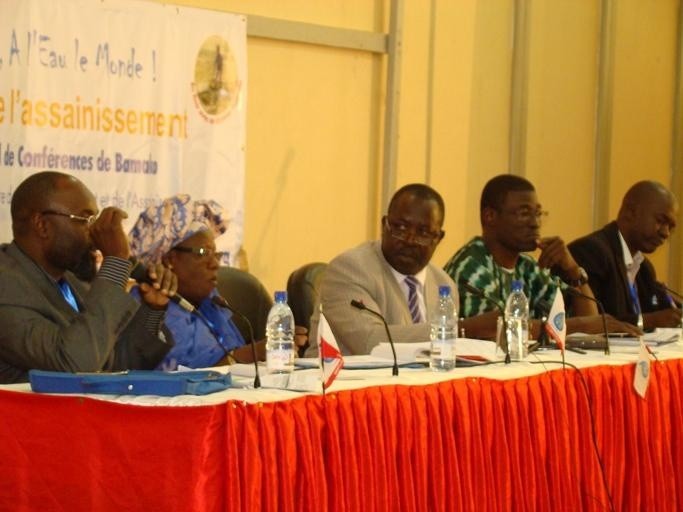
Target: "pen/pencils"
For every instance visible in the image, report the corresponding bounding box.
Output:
[602,334,640,337]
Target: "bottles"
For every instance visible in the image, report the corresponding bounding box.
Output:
[427,285,458,370]
[504,281,529,362]
[264,291,296,375]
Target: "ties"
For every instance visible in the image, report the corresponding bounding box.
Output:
[58,276,81,314]
[404,275,425,327]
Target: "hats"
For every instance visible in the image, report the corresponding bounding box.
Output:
[122,192,230,265]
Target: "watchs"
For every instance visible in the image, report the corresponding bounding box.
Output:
[565,266,587,286]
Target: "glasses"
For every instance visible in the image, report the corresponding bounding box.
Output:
[494,206,549,221]
[40,209,98,229]
[384,216,443,247]
[172,246,223,261]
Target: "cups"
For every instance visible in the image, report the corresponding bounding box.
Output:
[493,316,522,358]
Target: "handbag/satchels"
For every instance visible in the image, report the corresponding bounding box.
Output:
[29,368,232,395]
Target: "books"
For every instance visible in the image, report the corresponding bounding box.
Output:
[182,338,510,388]
[597,325,679,351]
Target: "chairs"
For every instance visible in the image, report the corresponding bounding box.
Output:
[206,263,271,348]
[285,261,330,360]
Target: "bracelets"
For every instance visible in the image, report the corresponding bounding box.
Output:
[226,347,238,367]
[459,315,466,337]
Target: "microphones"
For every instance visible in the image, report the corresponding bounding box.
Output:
[464,283,510,363]
[651,282,683,299]
[350,300,398,376]
[127,256,193,313]
[213,293,261,388]
[566,286,610,354]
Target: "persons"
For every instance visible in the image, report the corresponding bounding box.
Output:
[441,176,647,341]
[304,185,503,356]
[550,180,682,329]
[114,192,308,369]
[0,169,178,383]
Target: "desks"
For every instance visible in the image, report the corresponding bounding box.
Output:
[0,328,682,512]
